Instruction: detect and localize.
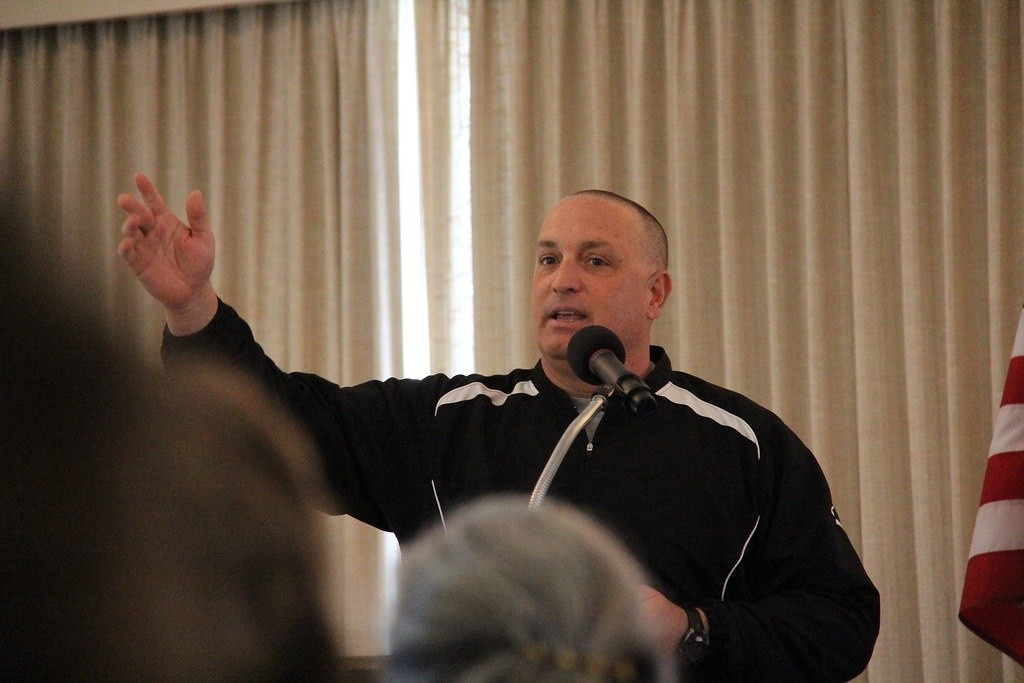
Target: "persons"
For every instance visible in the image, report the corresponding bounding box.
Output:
[109,169,887,683]
[0,251,680,683]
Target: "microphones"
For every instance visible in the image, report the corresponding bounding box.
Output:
[567,324,658,417]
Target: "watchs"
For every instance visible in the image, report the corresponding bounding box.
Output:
[676,603,709,667]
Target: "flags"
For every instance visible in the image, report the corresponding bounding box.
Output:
[958,299,1024,671]
